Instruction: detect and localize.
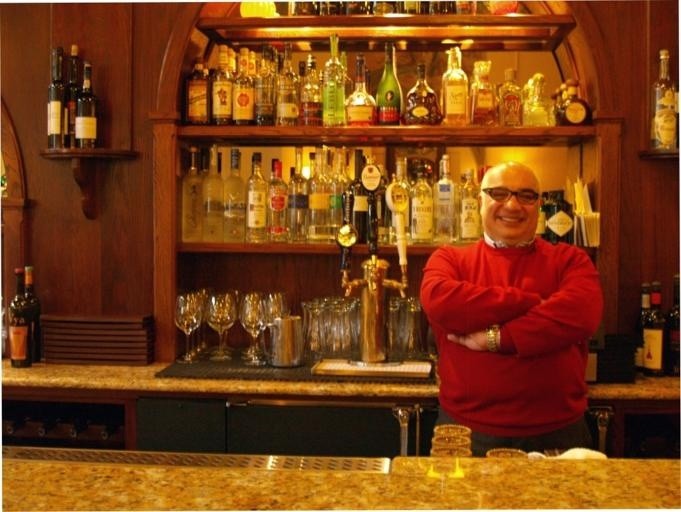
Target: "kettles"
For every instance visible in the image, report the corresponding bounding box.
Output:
[259,313,304,370]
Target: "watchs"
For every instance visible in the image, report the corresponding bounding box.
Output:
[484,321,502,354]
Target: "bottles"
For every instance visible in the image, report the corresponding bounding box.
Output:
[552,77,593,125]
[334,143,353,230]
[254,43,278,127]
[183,37,210,126]
[74,60,101,148]
[64,44,83,149]
[181,142,202,243]
[434,154,462,243]
[374,43,404,124]
[470,60,501,127]
[405,62,442,126]
[209,39,235,126]
[411,157,435,243]
[24,266,42,363]
[345,54,377,126]
[223,149,246,243]
[441,46,470,127]
[319,32,345,126]
[633,281,652,373]
[366,153,391,244]
[647,48,677,151]
[245,152,269,243]
[460,166,481,241]
[269,158,290,242]
[343,147,374,244]
[544,187,576,245]
[201,144,225,243]
[272,41,301,127]
[535,197,547,242]
[525,72,557,126]
[498,67,525,127]
[389,156,413,242]
[301,54,324,126]
[8,267,35,369]
[46,45,70,149]
[294,1,459,14]
[642,280,671,377]
[232,46,256,127]
[665,274,680,377]
[308,142,338,244]
[287,145,309,243]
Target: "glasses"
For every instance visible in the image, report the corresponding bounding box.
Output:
[482,187,541,203]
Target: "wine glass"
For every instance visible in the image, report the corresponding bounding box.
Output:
[170,289,289,367]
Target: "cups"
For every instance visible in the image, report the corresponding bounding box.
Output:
[301,298,442,365]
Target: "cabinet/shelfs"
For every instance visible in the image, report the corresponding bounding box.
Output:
[2,397,126,452]
[137,397,225,456]
[178,253,596,351]
[419,406,615,458]
[174,137,598,253]
[624,414,681,459]
[227,0,547,14]
[227,403,418,457]
[185,47,593,126]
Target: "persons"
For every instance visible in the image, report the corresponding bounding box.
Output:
[419,161,604,456]
